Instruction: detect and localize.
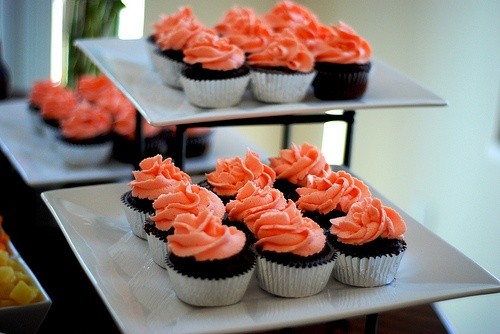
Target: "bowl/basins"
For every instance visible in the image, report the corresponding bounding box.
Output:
[0,228,53,334]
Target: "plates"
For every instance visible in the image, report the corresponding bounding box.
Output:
[40,164,500,334]
[74,35,449,128]
[0,98,274,187]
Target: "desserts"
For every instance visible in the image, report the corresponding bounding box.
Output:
[146,0,372,107]
[121,144,407,306]
[28,74,215,165]
